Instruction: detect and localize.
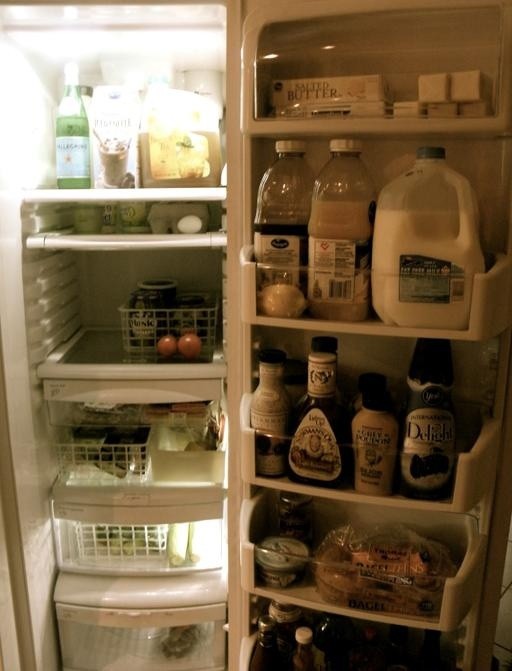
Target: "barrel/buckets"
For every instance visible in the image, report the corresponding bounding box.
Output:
[368,145,486,331]
[139,79,222,187]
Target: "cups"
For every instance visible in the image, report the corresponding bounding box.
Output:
[98,140,129,187]
[74,205,103,234]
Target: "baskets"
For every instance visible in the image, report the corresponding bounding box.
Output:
[53,424,152,483]
[117,292,219,353]
[75,523,169,562]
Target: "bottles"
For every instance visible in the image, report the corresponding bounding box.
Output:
[251,600,442,670]
[249,337,458,498]
[55,63,91,188]
[255,139,376,322]
